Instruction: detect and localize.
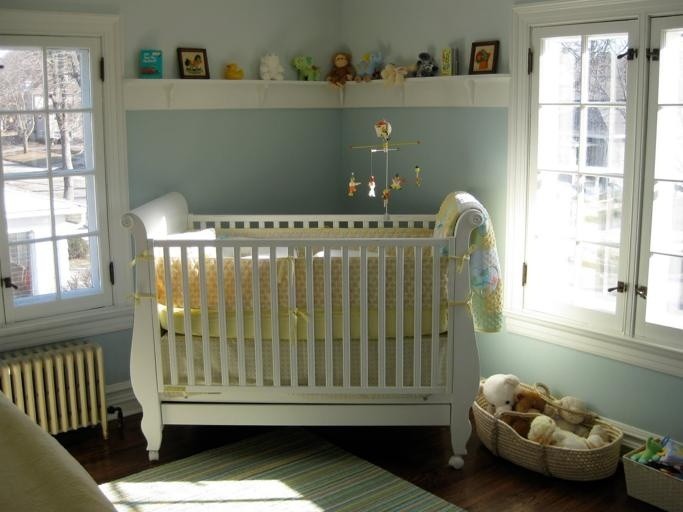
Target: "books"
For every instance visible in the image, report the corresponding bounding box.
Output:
[137,49,163,78]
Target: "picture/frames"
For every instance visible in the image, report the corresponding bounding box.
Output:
[468,40,499,75]
[177,48,210,79]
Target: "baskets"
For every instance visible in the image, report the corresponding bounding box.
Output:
[472,381,623,479]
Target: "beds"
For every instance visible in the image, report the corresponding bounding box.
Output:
[118,191,480,470]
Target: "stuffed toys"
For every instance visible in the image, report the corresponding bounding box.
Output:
[526,413,609,454]
[380,61,415,85]
[258,50,285,82]
[628,434,683,479]
[478,373,519,416]
[499,390,544,440]
[293,55,320,82]
[353,51,385,83]
[223,63,244,80]
[550,397,589,437]
[324,52,353,90]
[412,52,438,79]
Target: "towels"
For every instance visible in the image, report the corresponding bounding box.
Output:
[430,190,504,333]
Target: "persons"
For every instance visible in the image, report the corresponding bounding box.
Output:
[192,54,202,72]
[184,59,192,70]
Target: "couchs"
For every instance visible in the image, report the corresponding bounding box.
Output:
[0,393,117,512]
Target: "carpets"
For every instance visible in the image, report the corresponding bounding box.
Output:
[100,435,471,512]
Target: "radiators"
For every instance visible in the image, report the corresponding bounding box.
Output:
[0,339,108,442]
